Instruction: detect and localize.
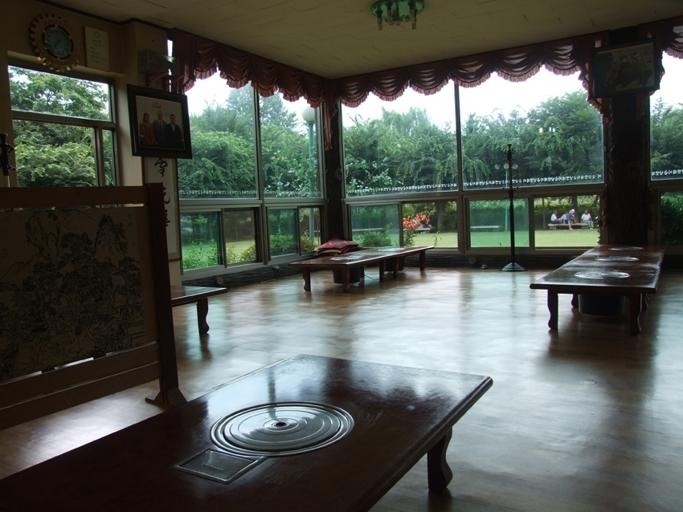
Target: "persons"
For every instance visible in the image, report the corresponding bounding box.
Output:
[138,112,156,146]
[580,209,594,230]
[166,113,183,148]
[550,210,561,229]
[560,208,576,231]
[150,109,169,148]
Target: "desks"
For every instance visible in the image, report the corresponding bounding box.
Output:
[288,246,435,294]
[529,245,667,335]
[0,353,494,512]
[170,285,228,336]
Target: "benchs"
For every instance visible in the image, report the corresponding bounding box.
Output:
[403,228,431,233]
[352,228,384,234]
[470,225,502,232]
[549,223,588,230]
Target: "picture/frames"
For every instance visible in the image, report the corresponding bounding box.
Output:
[126,83,193,159]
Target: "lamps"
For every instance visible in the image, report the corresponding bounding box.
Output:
[368,0,424,31]
